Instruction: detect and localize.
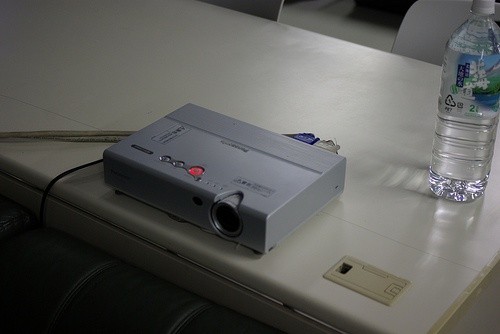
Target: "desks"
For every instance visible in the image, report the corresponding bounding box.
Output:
[1,0,500,334]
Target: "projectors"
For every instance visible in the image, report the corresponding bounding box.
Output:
[102,103,347,255]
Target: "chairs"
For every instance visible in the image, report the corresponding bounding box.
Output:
[391,0,500,66]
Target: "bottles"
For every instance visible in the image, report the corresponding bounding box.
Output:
[428,0,500,202]
